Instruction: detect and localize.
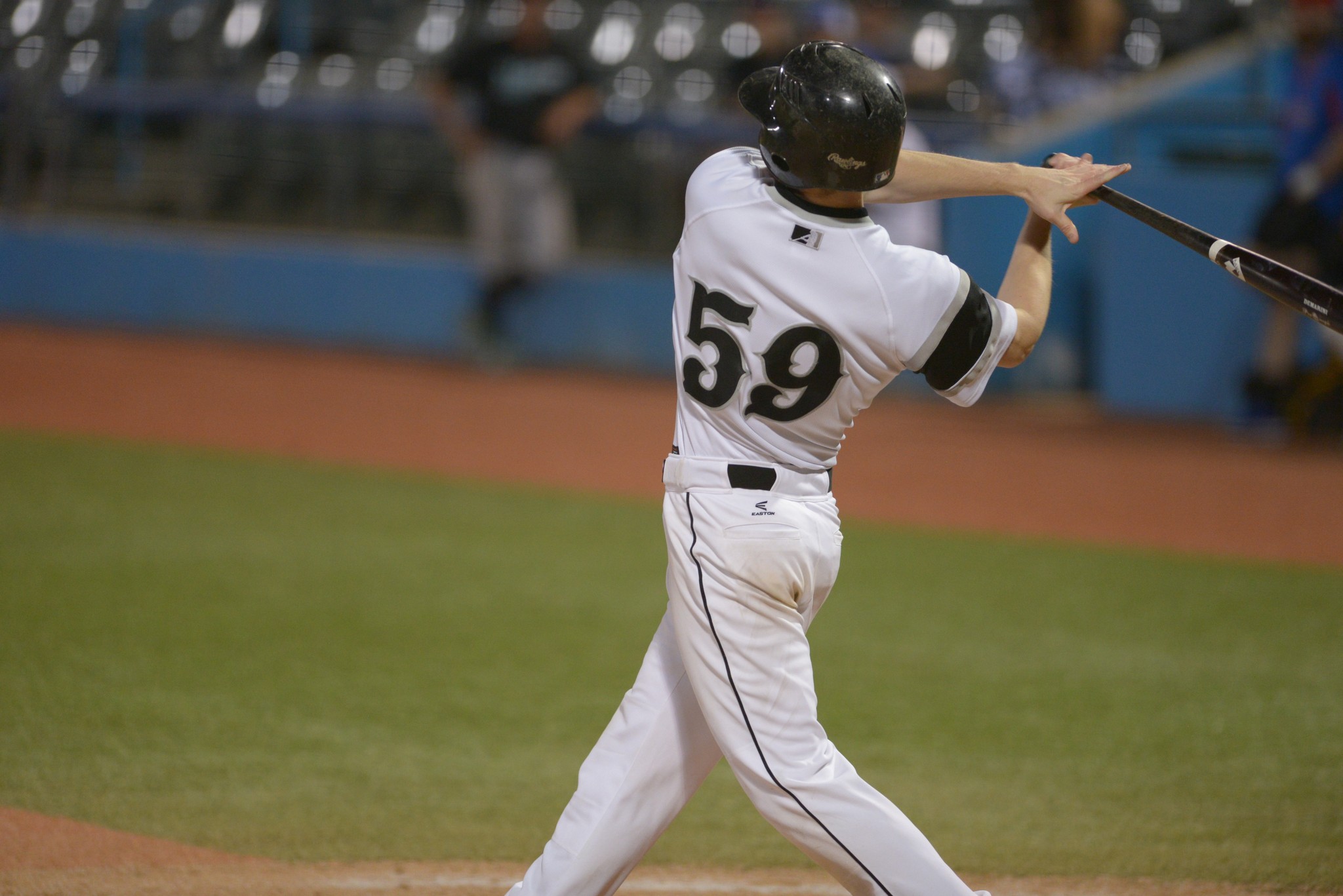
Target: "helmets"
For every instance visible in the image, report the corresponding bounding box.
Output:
[737,39,906,191]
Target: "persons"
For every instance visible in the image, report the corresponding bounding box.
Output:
[1235,0,1342,421]
[500,41,1132,896]
[419,0,606,365]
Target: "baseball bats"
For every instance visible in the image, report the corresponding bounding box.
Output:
[1038,132,1343,364]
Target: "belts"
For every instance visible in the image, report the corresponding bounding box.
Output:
[662,459,832,495]
